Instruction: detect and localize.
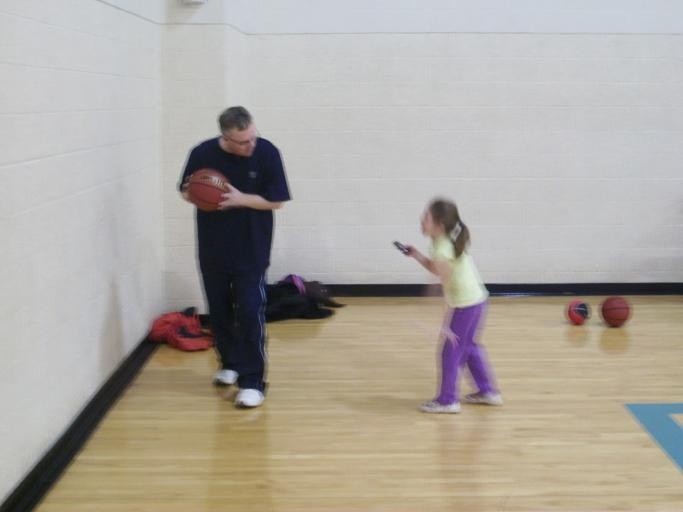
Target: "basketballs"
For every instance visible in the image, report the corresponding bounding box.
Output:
[602,297,629,326]
[188,169,228,212]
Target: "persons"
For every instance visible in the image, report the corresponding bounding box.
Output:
[178,105,289,408]
[402,201,503,414]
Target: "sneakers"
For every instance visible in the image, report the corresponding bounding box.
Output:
[210,368,239,386]
[233,388,264,409]
[416,397,462,415]
[461,391,506,407]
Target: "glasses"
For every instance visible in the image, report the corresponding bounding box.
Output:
[224,127,261,147]
